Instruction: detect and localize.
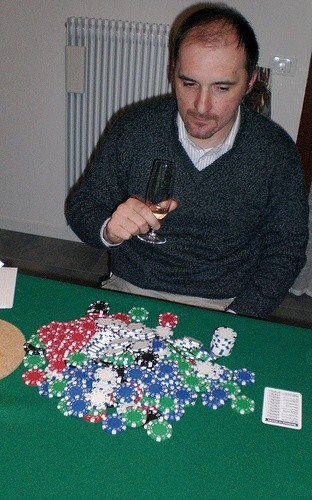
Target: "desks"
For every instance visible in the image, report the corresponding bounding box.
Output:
[0,272,312,500]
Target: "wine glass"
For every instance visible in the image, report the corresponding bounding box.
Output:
[137,159,176,244]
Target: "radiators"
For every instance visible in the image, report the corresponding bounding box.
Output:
[64,16,178,198]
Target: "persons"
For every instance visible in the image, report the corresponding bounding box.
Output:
[64,7,308,319]
